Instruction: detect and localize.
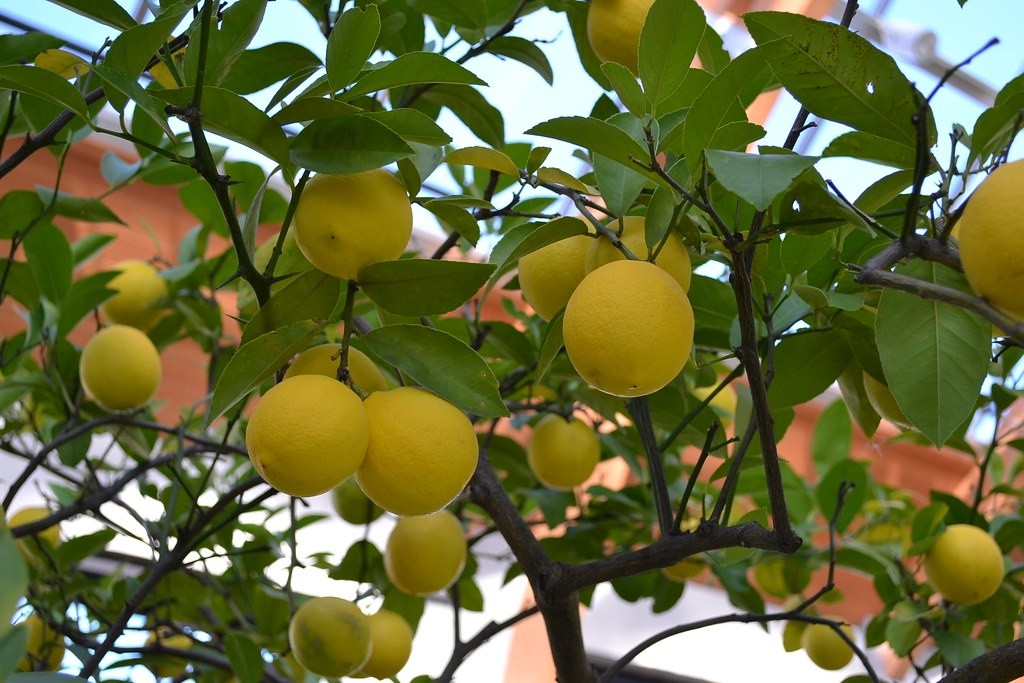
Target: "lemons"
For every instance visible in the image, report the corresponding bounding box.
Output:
[78,0,1024,681]
[4,508,65,672]
